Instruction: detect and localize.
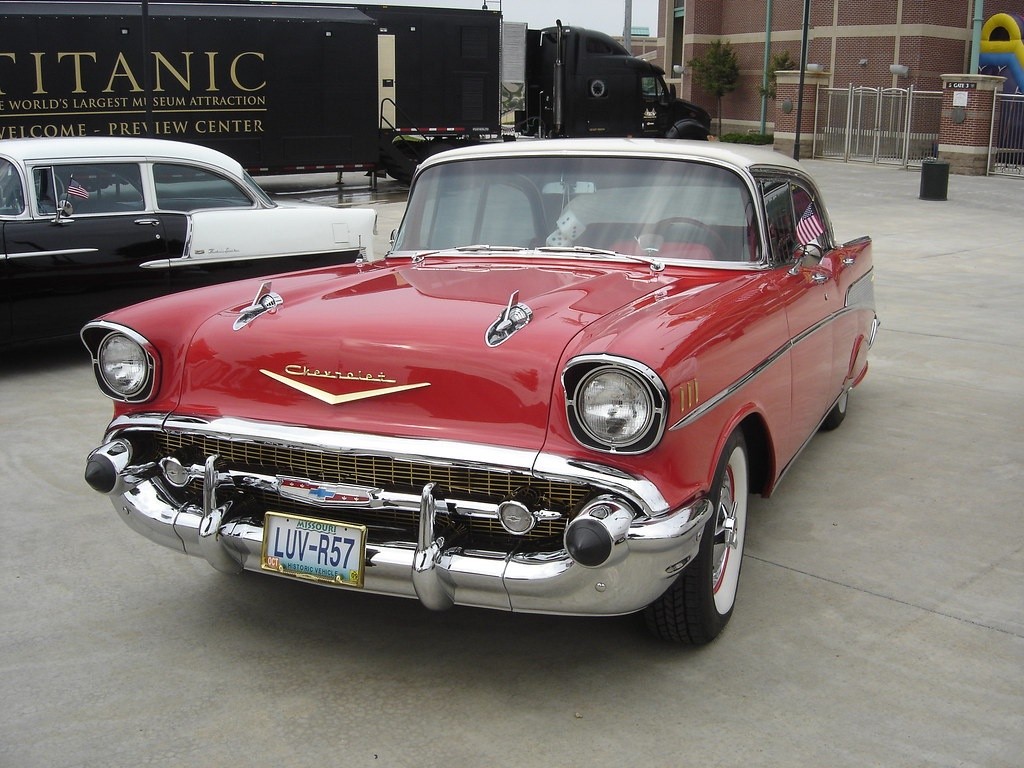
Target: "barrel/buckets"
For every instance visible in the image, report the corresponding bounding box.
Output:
[918,160,951,201]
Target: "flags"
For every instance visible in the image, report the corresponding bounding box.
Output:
[67,178,89,199]
[795,204,826,247]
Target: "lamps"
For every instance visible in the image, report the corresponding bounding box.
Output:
[889,64,909,79]
[672,64,689,76]
[807,63,825,72]
[858,59,869,65]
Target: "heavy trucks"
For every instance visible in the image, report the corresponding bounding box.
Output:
[336,2,713,188]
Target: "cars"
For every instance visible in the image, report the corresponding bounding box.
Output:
[0,135,378,356]
[78,131,881,648]
